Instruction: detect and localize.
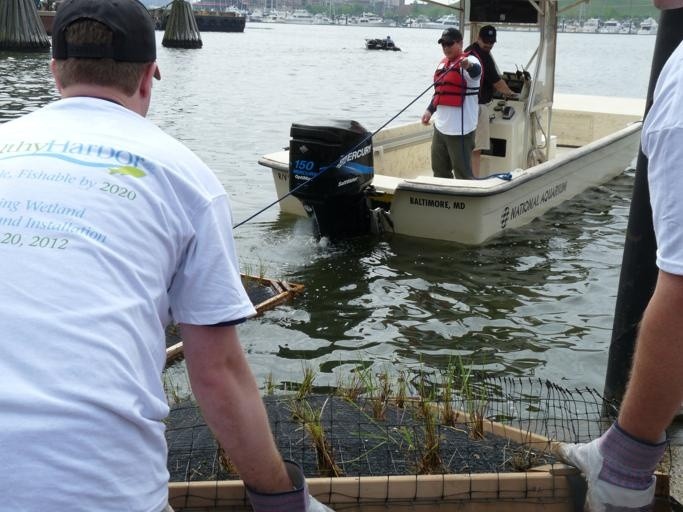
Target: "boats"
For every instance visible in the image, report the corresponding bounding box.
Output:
[258,0,650,249]
[363,38,401,53]
[557,15,660,35]
[221,1,462,31]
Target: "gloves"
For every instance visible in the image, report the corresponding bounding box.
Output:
[243,459,337,512]
[550,420,670,512]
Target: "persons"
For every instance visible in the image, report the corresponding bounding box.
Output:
[0,1,335,512]
[422,27,483,179]
[551,2,683,511]
[464,25,516,179]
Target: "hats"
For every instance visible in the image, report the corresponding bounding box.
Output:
[479,25,496,43]
[50,0,162,81]
[438,27,464,45]
